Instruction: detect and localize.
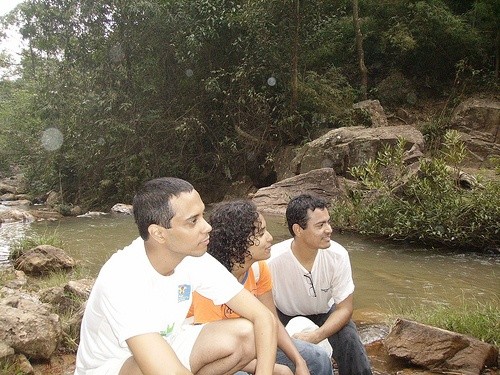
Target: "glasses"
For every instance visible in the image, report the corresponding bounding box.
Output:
[304,272,317,297]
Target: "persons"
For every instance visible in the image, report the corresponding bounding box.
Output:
[186,200,334,375]
[264,193,372,375]
[73,176,279,375]
